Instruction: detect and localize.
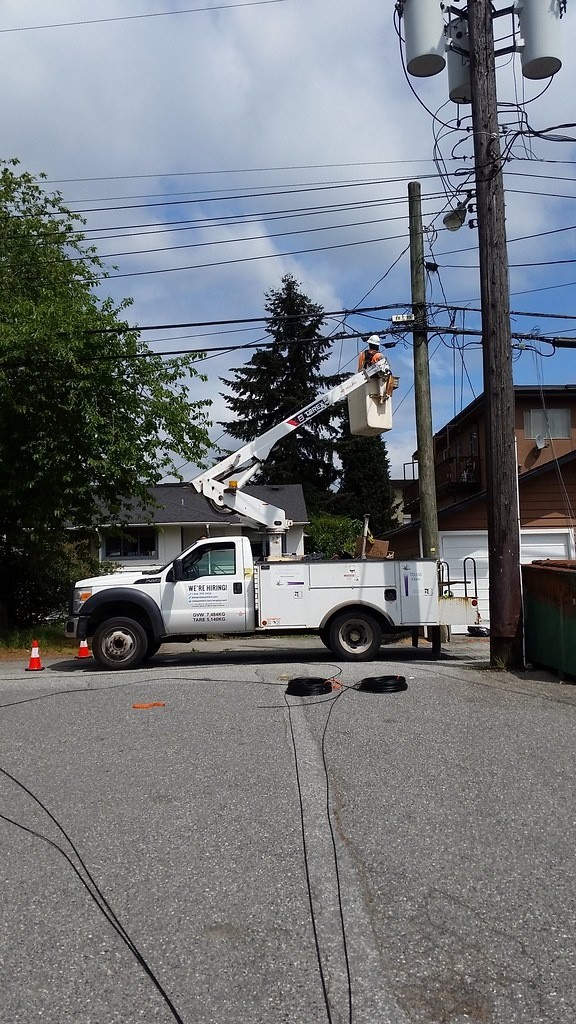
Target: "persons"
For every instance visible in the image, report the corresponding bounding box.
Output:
[358,335,390,374]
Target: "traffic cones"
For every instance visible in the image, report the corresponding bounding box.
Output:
[25,640,45,671]
[74,638,93,659]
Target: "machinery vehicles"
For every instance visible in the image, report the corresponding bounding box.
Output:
[64,358,482,671]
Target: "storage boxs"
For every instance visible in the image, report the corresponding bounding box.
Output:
[354,536,390,560]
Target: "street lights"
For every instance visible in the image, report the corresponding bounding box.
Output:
[443,192,526,670]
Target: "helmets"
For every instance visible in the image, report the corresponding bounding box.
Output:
[367,335,381,346]
